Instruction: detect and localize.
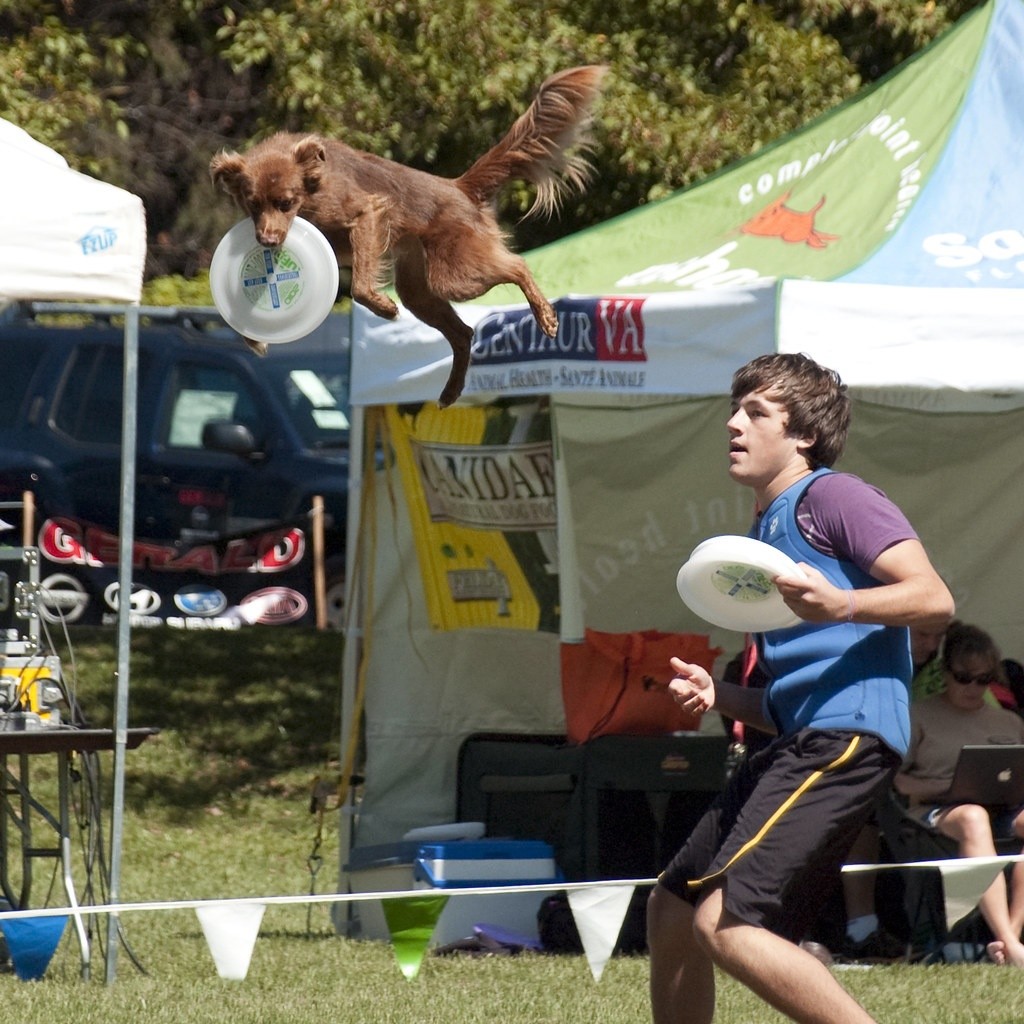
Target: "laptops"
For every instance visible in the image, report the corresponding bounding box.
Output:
[919,746,1024,807]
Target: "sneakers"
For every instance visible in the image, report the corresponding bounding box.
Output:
[839,928,929,965]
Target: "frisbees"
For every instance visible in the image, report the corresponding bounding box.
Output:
[209,215,340,345]
[675,535,806,632]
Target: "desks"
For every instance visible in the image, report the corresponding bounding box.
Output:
[0,727,164,984]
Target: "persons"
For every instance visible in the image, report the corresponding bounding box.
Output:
[646,352,954,1024]
[841,622,1001,964]
[891,623,1024,967]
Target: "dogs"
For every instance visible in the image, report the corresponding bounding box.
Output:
[211,65,610,412]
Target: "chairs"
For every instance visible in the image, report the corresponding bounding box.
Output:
[874,786,1024,967]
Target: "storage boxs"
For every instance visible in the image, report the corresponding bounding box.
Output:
[413,838,568,955]
[342,839,420,943]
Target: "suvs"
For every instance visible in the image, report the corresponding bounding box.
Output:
[0,298,382,631]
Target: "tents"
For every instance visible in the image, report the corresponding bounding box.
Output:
[0,115,147,982]
[336,0,1024,936]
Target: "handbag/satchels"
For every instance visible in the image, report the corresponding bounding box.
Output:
[559,629,718,744]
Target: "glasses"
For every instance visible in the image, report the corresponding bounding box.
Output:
[946,665,994,685]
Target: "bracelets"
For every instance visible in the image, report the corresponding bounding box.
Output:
[847,589,854,621]
